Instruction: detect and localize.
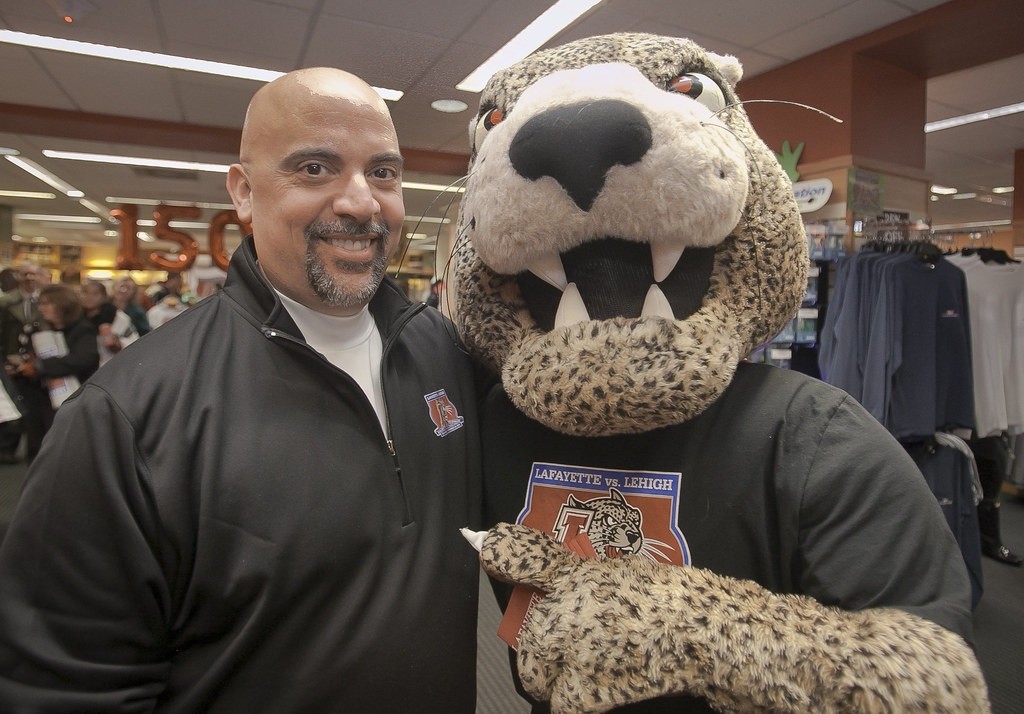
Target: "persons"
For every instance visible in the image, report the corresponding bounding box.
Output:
[0,66,488,714]
[0,261,223,465]
[425,275,444,308]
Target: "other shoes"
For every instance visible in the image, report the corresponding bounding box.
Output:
[0,454,22,465]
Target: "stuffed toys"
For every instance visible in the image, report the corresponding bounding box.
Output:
[446,30,991,714]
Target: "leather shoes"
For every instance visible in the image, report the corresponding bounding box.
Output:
[983,545,1024,565]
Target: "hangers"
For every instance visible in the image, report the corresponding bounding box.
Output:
[861,216,1002,254]
